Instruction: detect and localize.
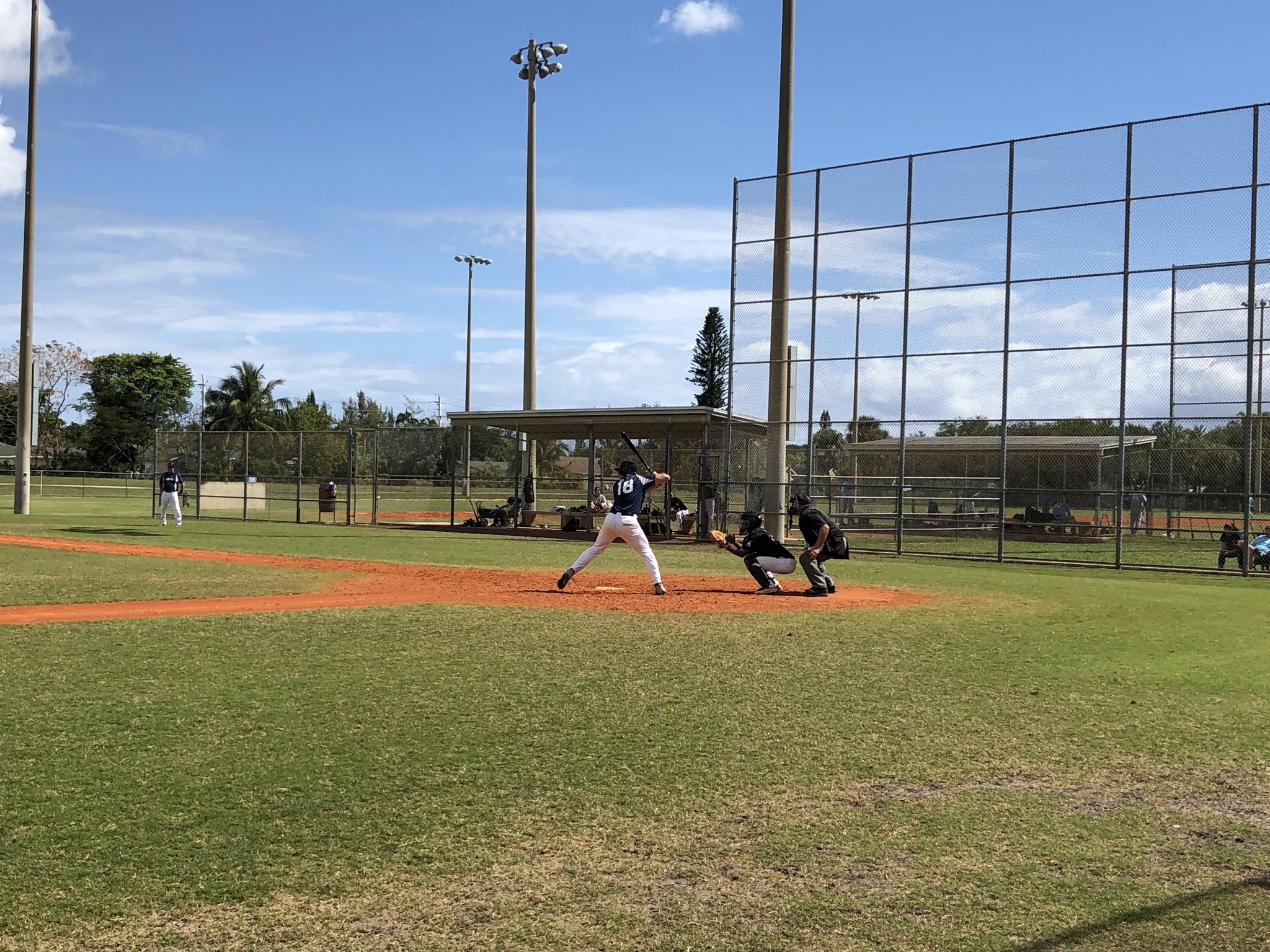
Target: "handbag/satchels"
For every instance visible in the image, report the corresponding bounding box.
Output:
[832,538,849,560]
[825,535,844,555]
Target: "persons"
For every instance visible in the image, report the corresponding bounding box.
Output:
[719,511,797,595]
[1230,520,1270,571]
[1218,523,1244,569]
[1040,490,1055,514]
[590,487,609,513]
[671,492,689,534]
[699,469,716,533]
[557,461,670,595]
[1124,487,1147,535]
[1241,496,1255,518]
[838,479,856,525]
[159,462,185,527]
[1052,498,1071,532]
[963,491,980,516]
[787,493,849,597]
[490,497,515,527]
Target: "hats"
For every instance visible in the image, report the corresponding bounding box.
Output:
[789,493,811,506]
[167,462,174,467]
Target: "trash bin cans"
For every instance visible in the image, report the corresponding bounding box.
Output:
[319,484,337,512]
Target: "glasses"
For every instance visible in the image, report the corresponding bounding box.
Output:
[168,466,174,468]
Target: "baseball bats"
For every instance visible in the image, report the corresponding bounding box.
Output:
[621,431,661,485]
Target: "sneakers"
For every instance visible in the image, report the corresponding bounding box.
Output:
[654,583,667,595]
[557,569,574,589]
[805,587,829,596]
[754,583,784,594]
[827,584,837,594]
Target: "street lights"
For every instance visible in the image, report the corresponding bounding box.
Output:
[454,254,494,496]
[1241,297,1270,514]
[843,291,880,503]
[510,38,567,511]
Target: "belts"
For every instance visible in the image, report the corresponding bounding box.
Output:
[610,510,634,516]
[163,490,177,492]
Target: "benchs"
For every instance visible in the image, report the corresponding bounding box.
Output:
[1005,518,1090,535]
[523,509,695,536]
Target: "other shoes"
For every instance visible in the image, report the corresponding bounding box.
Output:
[159,523,166,527]
[175,524,181,527]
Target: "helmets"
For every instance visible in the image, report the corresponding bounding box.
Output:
[613,461,637,475]
[738,511,763,536]
